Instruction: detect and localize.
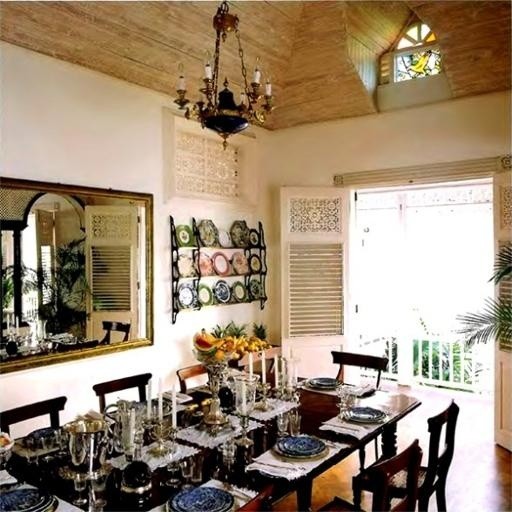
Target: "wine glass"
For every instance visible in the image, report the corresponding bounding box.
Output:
[25,400,183,508]
[276,412,290,438]
[274,351,301,404]
[233,373,257,448]
[180,453,204,484]
[1,315,83,360]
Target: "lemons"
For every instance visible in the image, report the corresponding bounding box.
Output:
[215,350,224,361]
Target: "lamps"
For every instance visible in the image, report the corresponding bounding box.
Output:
[172,0,276,147]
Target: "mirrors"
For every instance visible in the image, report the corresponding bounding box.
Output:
[0,175,155,376]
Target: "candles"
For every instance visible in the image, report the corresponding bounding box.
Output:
[5,312,21,337]
[143,387,178,429]
[243,347,282,387]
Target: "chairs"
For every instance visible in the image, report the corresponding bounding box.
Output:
[345,398,461,512]
[0,394,69,446]
[327,348,389,471]
[236,485,283,512]
[90,372,151,412]
[96,317,132,349]
[230,341,284,381]
[174,362,212,395]
[310,437,425,512]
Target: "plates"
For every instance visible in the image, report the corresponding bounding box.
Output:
[166,487,234,512]
[271,435,330,460]
[173,219,261,309]
[304,377,344,389]
[0,487,59,511]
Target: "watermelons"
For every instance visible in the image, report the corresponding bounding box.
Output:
[194,335,216,353]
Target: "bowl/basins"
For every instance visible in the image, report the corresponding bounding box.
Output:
[342,405,386,424]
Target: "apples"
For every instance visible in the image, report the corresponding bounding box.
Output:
[226,336,272,360]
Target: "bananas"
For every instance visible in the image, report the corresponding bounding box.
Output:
[201,329,225,348]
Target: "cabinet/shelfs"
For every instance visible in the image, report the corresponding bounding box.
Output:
[169,213,269,325]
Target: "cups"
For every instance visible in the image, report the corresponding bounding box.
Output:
[335,386,357,418]
[221,456,234,474]
[216,436,237,457]
[287,416,303,437]
